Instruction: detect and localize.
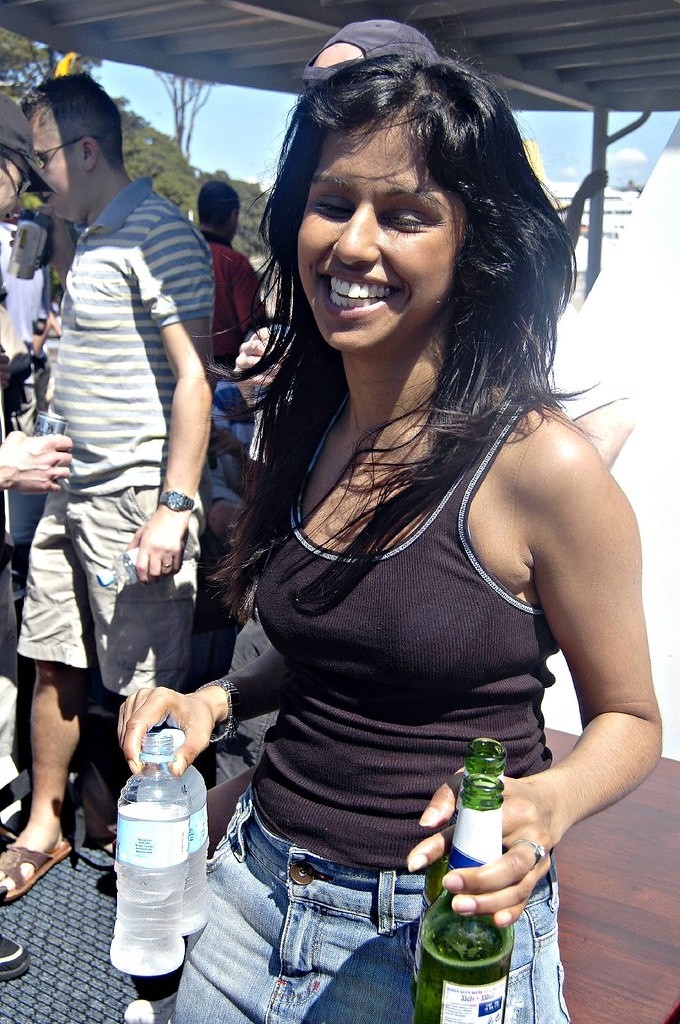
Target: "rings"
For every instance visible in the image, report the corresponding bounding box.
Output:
[509,837,545,869]
[162,563,172,568]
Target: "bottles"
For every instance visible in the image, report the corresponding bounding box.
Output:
[248,325,297,463]
[137,729,210,936]
[207,423,218,471]
[110,732,185,977]
[412,738,516,1024]
[95,530,200,587]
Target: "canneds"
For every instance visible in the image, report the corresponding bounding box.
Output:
[31,410,69,437]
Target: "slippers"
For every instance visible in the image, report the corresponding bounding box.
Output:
[0,838,73,902]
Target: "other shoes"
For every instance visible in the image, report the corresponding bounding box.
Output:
[0,934,31,981]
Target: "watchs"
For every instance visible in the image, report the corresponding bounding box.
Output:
[158,491,194,512]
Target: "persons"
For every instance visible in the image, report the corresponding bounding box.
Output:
[115,54,663,1024]
[0,19,607,980]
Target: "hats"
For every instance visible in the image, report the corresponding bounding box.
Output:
[0,92,33,179]
[301,19,440,88]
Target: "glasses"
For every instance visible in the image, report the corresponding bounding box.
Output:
[31,135,101,169]
[7,175,32,198]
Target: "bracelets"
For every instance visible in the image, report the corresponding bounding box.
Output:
[195,679,240,744]
